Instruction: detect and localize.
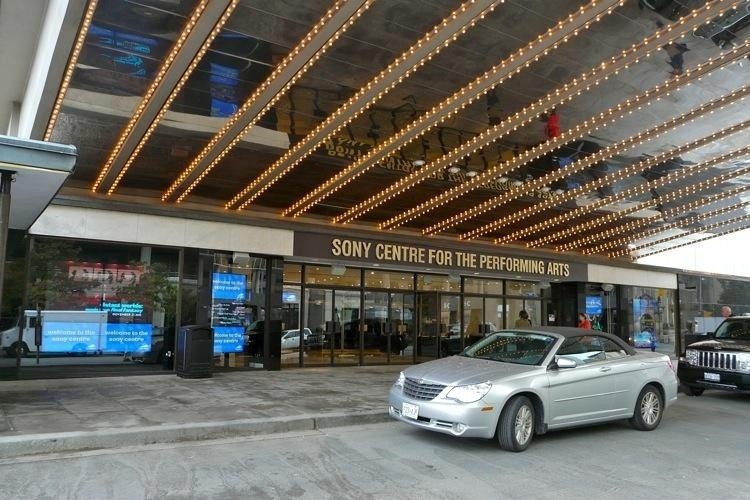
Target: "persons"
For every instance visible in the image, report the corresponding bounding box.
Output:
[720,305,743,334]
[485,92,502,125]
[663,42,689,76]
[515,310,531,328]
[539,107,562,138]
[577,313,592,329]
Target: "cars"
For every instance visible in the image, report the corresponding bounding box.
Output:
[387,326,679,453]
[337,316,407,354]
[306,330,340,349]
[281,327,313,351]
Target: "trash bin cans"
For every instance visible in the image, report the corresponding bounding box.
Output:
[176,325,215,379]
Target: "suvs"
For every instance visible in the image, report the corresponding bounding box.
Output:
[678,313,750,397]
